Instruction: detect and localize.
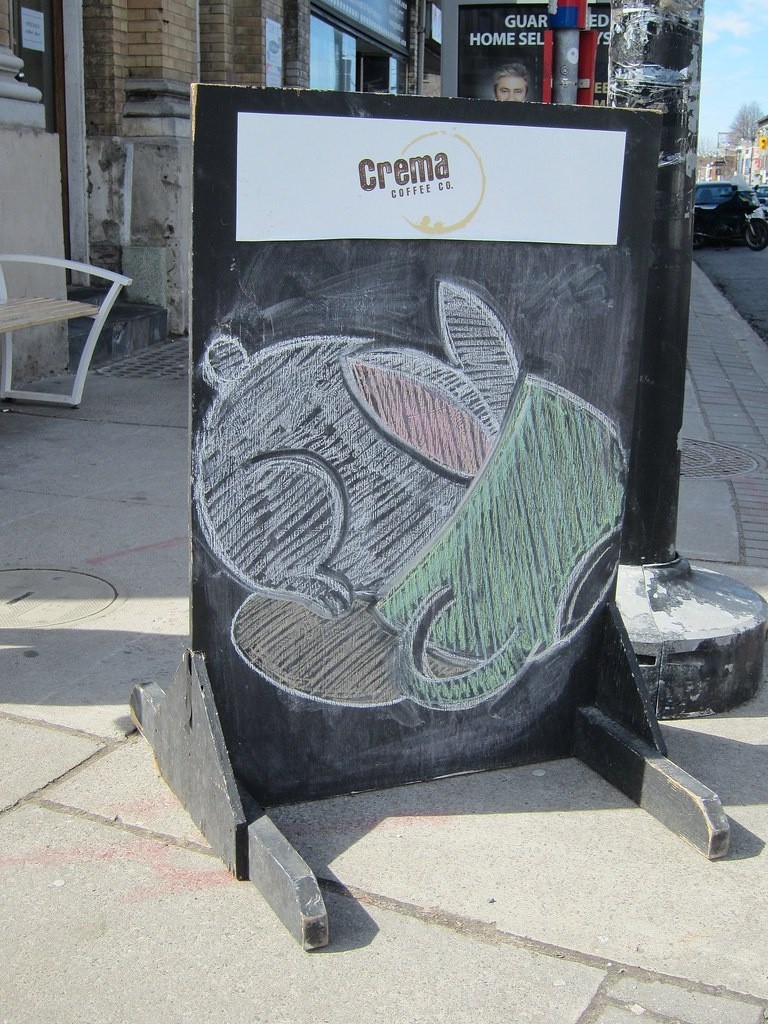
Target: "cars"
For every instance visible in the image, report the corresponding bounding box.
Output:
[695,180,734,208]
[750,185,768,219]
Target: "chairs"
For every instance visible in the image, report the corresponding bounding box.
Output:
[699,189,713,203]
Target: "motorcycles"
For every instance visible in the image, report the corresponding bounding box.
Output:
[694,185,768,251]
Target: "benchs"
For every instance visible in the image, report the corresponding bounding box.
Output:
[0,255,133,408]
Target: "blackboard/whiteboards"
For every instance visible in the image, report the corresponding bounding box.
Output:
[185,81,670,810]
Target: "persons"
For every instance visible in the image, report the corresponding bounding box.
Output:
[491,63,530,101]
